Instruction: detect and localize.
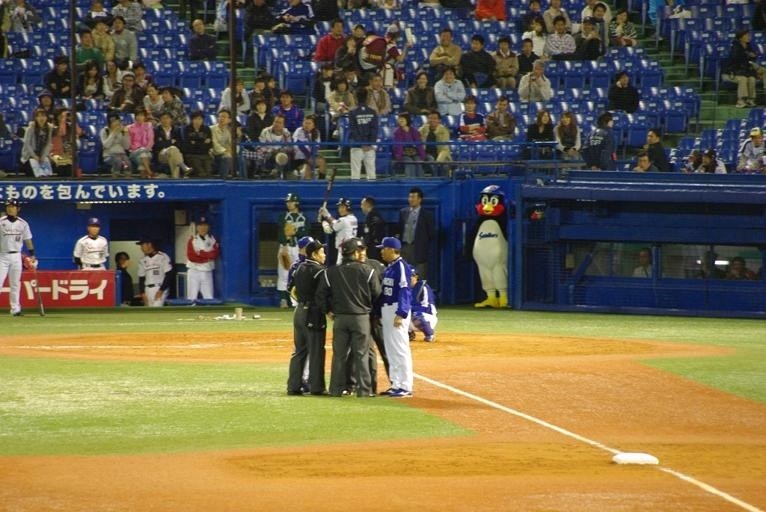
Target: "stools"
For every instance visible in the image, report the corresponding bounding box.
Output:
[176,271,187,299]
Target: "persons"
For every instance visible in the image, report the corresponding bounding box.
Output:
[0,198,35,316]
[633,249,652,278]
[136,237,175,306]
[115,251,133,304]
[72,218,110,271]
[277,188,438,397]
[185,216,220,300]
[723,256,756,281]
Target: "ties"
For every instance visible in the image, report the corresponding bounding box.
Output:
[406,211,415,245]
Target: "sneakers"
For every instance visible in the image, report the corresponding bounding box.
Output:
[379,389,397,396]
[422,333,437,343]
[144,284,155,288]
[390,387,415,398]
[81,263,104,268]
[287,378,377,398]
[280,298,290,309]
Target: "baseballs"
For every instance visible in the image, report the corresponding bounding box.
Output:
[343,390,348,394]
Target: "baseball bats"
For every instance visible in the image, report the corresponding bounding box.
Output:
[320,168,337,216]
[32,261,45,316]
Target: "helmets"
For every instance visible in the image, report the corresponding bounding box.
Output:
[285,192,300,205]
[335,197,352,207]
[3,197,21,208]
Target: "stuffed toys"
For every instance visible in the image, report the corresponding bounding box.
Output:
[471,185,508,308]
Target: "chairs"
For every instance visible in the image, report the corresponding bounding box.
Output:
[0,0,766,171]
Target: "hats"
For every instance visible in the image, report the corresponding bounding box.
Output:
[86,217,101,228]
[373,236,402,250]
[349,237,369,250]
[304,240,328,253]
[134,231,158,245]
[748,126,763,136]
[298,235,314,250]
[341,240,360,256]
[697,149,716,160]
[195,216,210,226]
